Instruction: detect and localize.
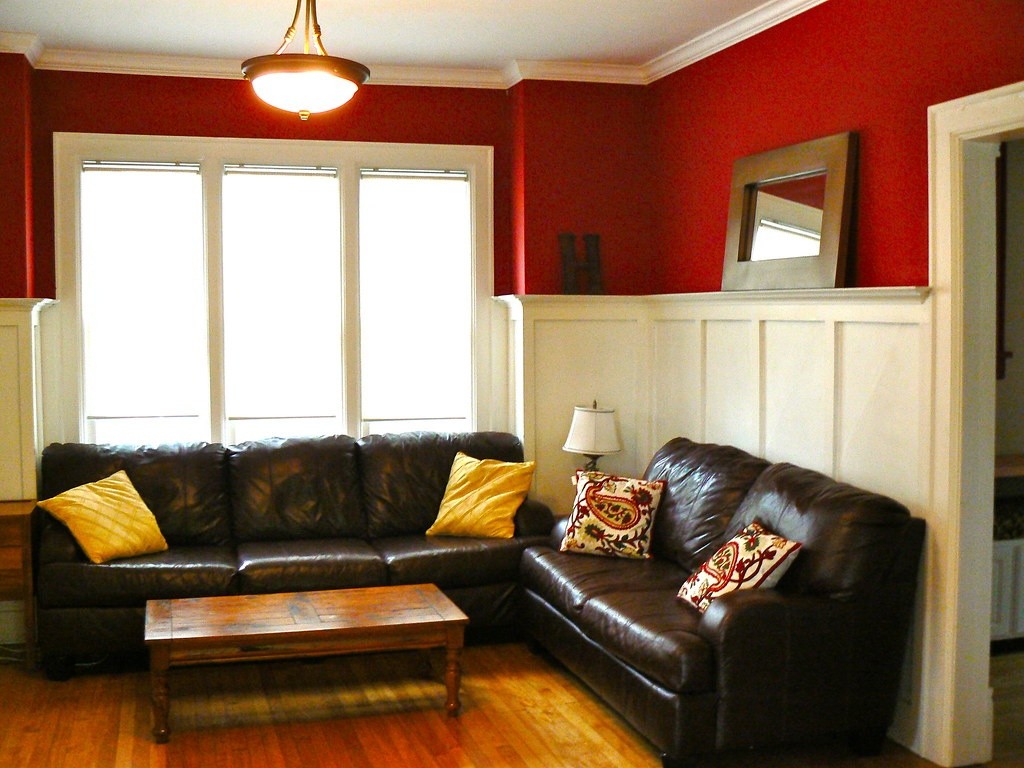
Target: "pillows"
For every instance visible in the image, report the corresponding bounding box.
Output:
[676,515,804,613]
[426,451,538,540]
[36,468,171,564]
[559,468,667,559]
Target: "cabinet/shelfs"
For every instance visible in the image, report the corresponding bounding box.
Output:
[990,538,1023,656]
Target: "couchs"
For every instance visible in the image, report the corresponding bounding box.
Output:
[30,428,559,679]
[518,439,925,762]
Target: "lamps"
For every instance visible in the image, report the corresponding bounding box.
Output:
[561,399,622,472]
[240,0,370,125]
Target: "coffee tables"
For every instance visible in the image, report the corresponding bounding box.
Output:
[141,581,471,746]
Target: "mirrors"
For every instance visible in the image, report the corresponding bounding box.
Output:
[718,130,858,291]
